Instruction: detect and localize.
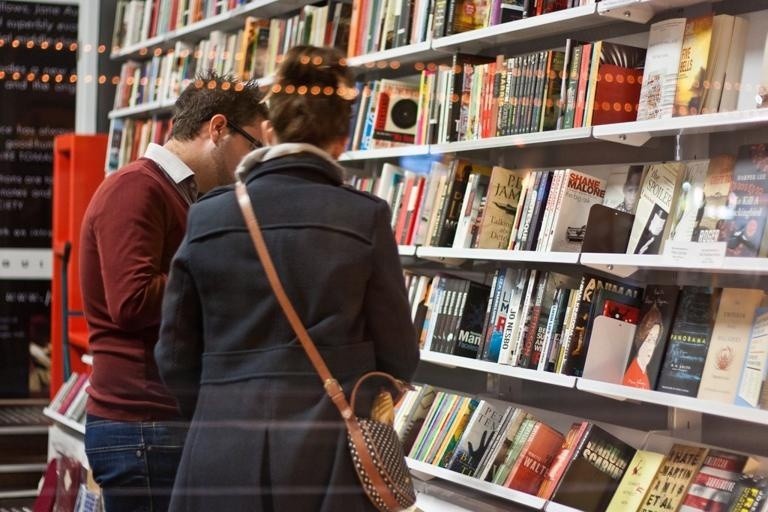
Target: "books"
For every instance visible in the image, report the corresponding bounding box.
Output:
[421,258,768,414]
[345,160,421,244]
[109,0,300,108]
[395,258,422,323]
[347,55,421,152]
[427,0,586,42]
[421,382,768,512]
[415,133,768,258]
[423,12,767,147]
[390,375,422,459]
[300,0,427,57]
[104,114,170,173]
[110,0,266,51]
[48,362,88,423]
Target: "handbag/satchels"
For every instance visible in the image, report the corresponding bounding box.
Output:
[345,371,416,512]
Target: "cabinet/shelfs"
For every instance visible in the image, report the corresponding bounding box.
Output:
[43,0,768,512]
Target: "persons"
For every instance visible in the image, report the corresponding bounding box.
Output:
[157,45,421,512]
[80,71,267,511]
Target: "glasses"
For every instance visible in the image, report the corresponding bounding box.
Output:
[227,121,265,151]
